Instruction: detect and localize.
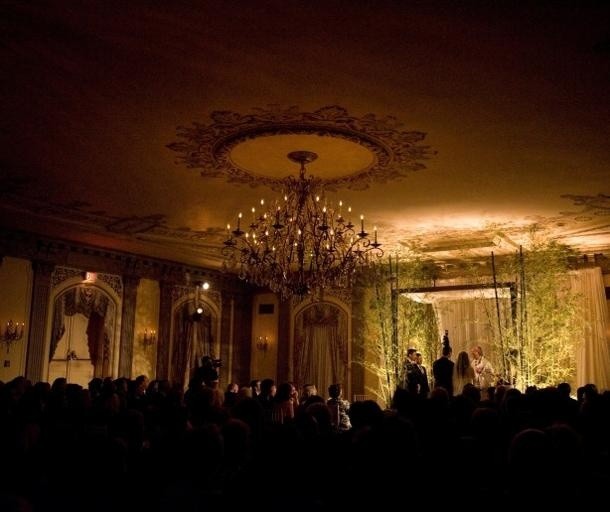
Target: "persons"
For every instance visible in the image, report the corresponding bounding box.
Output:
[1,375,609,512]
[404,349,422,396]
[189,355,219,388]
[452,351,475,396]
[432,345,454,395]
[471,345,497,391]
[415,353,431,393]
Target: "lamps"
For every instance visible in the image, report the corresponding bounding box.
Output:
[7,322,24,336]
[257,335,267,349]
[226,151,377,303]
[144,330,156,340]
